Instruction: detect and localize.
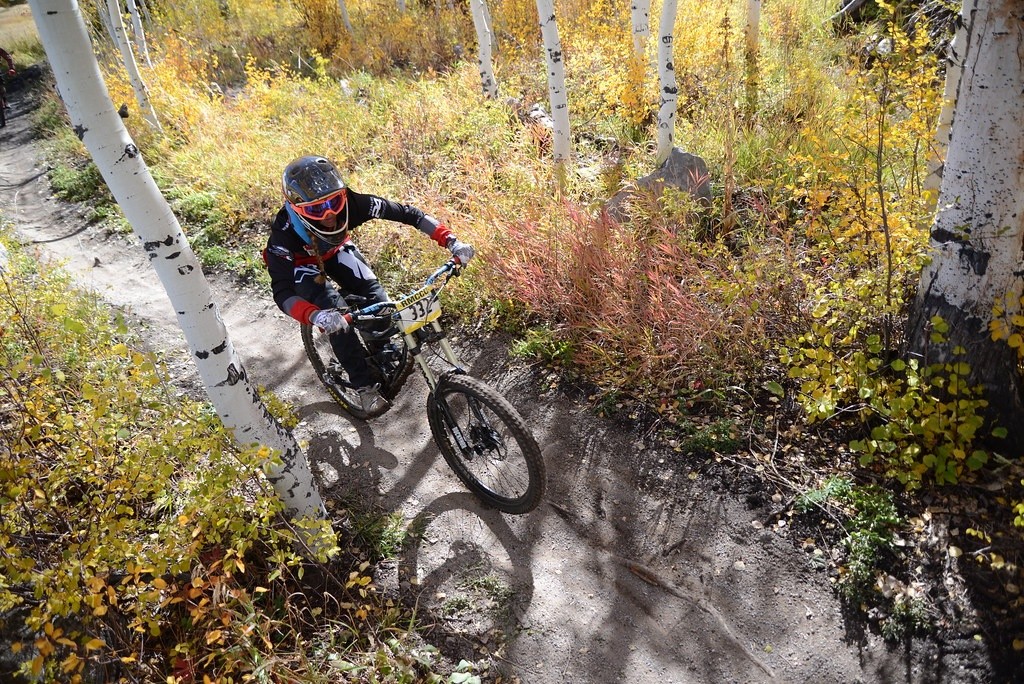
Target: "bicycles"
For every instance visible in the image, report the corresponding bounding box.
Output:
[300,256,548,516]
[0,71,12,129]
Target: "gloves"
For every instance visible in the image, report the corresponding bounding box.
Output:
[309,306,349,338]
[446,236,471,270]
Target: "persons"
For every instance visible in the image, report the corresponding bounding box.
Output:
[0,47,15,128]
[263,155,475,417]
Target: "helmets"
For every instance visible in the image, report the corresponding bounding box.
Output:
[282,157,348,243]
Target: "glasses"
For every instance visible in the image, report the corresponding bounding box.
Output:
[302,189,346,220]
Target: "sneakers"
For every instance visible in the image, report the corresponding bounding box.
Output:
[356,383,388,414]
